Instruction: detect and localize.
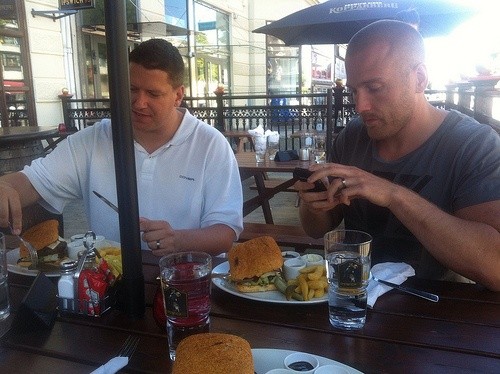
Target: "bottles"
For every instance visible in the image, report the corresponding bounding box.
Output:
[60,258,76,310]
[77,250,95,273]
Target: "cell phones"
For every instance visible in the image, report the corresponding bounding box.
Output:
[294,167,327,193]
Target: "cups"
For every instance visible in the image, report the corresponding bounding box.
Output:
[0,231,10,319]
[255,136,266,162]
[265,351,348,374]
[300,146,310,161]
[269,142,279,159]
[159,252,213,361]
[67,234,105,259]
[323,229,372,331]
[280,250,324,281]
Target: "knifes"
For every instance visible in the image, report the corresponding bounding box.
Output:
[93,190,118,214]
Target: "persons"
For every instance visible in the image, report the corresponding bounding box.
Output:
[294,19,500,293]
[0,39,244,259]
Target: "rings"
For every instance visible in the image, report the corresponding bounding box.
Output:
[157,240,160,249]
[342,176,347,189]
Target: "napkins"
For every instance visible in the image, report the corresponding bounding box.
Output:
[264,129,280,145]
[367,261,416,310]
[90,355,129,374]
[248,125,267,155]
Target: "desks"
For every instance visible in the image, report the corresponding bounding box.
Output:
[224,130,256,152]
[0,123,70,239]
[288,130,327,150]
[235,151,325,224]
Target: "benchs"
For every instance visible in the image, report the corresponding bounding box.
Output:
[238,223,345,253]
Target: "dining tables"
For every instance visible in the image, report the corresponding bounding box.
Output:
[0,234,500,374]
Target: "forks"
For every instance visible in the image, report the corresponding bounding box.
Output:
[9,221,38,265]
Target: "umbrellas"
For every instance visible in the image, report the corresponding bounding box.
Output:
[251,0,471,45]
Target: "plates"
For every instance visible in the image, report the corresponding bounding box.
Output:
[251,348,364,374]
[6,240,119,277]
[212,258,329,305]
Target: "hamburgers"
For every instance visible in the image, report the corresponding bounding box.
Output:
[227,235,284,292]
[17,220,68,267]
[172,332,256,374]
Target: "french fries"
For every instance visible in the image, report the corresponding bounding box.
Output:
[286,264,330,302]
[91,247,123,265]
